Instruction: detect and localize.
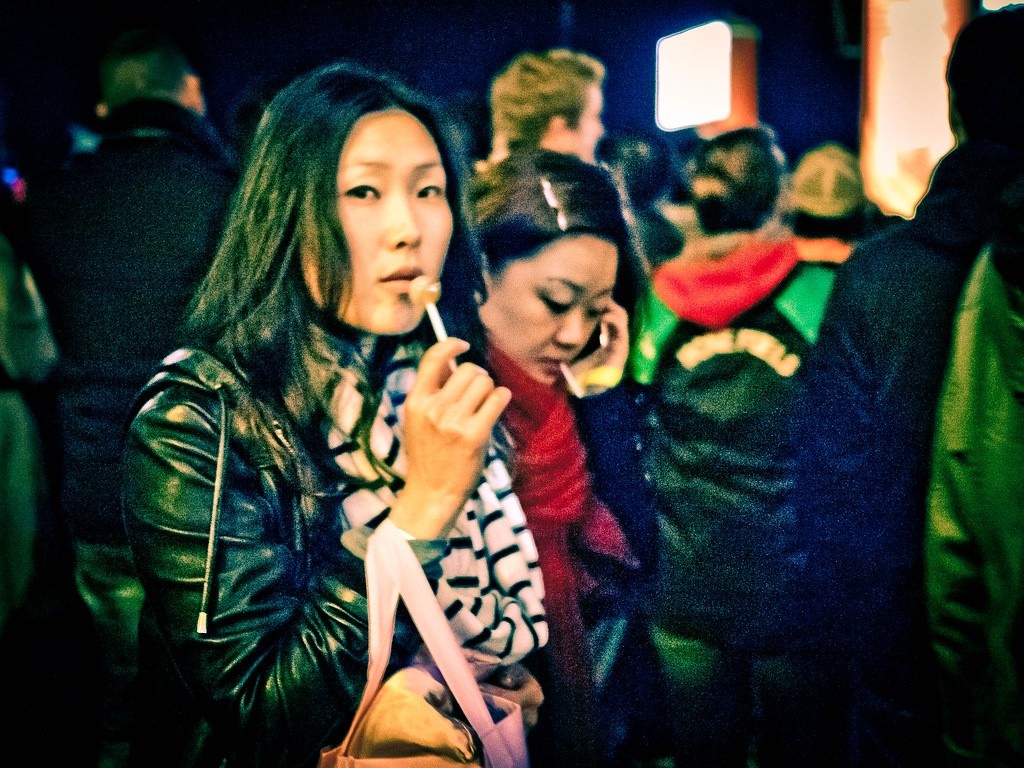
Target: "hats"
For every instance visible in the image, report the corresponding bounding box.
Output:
[789,144,863,219]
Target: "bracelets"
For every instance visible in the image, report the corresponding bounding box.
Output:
[574,365,620,387]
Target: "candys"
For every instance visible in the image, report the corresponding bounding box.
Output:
[408,274,457,373]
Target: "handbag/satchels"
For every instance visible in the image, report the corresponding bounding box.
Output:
[318,525,528,768]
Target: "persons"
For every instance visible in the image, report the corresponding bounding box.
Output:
[629,127,836,768]
[0,8,1024,768]
[469,147,660,768]
[24,40,268,767]
[118,62,549,768]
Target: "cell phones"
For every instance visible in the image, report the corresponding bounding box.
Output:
[570,324,613,371]
